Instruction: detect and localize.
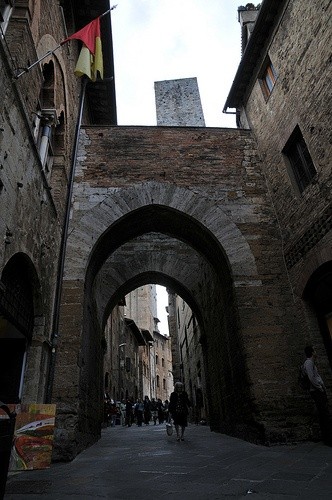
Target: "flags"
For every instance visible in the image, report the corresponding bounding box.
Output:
[60,15,103,83]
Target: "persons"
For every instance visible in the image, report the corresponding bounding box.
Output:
[167,382,193,442]
[303,345,332,448]
[103,392,171,427]
